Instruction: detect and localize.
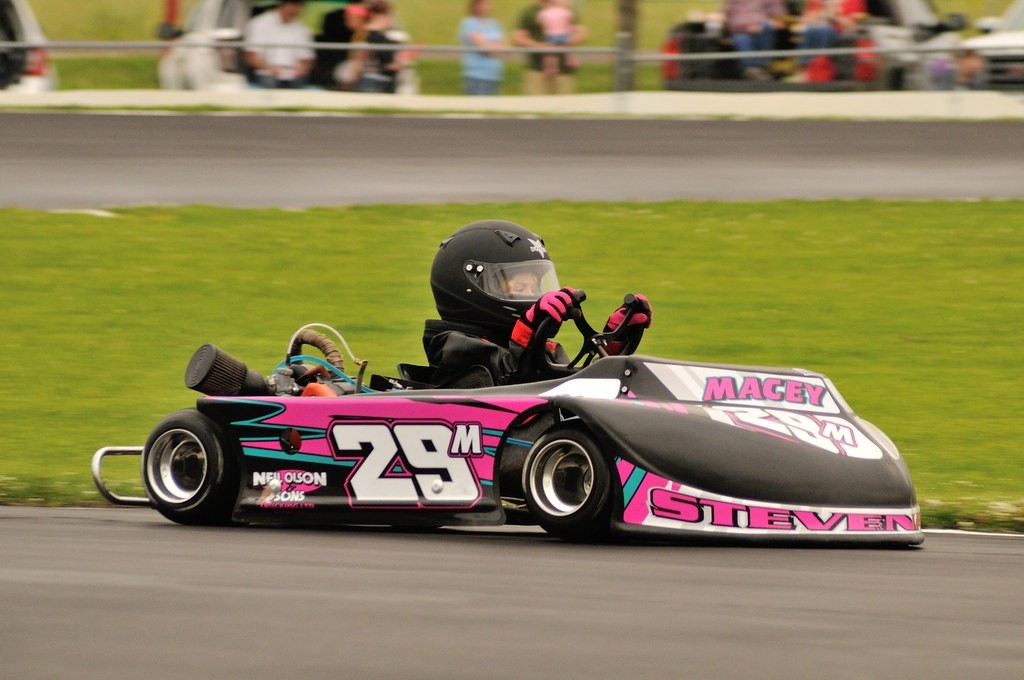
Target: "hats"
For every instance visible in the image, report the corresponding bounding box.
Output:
[345,6,368,17]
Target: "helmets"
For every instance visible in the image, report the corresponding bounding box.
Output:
[430,220,561,350]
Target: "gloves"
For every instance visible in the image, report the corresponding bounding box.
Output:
[524,287,577,339]
[602,294,653,355]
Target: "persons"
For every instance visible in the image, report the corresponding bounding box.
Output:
[242,0,411,92]
[913,15,999,89]
[423,220,652,389]
[720,0,876,82]
[457,0,509,95]
[511,0,584,95]
